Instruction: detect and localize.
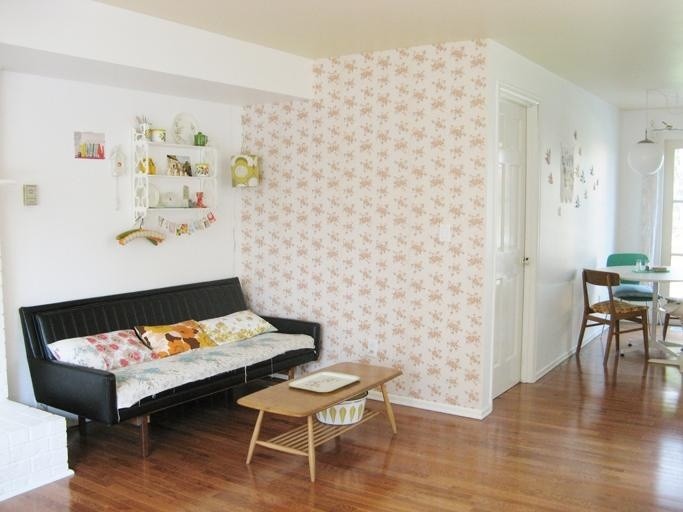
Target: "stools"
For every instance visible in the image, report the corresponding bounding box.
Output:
[0,400,75,506]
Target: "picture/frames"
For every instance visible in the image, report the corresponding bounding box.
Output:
[109,143,127,178]
[74,131,106,160]
[23,185,38,206]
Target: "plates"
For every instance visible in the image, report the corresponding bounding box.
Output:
[289,371,360,394]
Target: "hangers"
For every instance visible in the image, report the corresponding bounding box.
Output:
[116,216,167,246]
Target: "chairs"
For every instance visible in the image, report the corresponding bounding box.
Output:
[600,253,663,339]
[662,297,683,343]
[575,269,649,366]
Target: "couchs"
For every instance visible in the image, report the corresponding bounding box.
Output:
[18,277,322,459]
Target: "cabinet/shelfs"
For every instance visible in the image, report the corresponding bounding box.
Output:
[130,127,217,222]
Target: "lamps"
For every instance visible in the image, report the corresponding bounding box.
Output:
[629,85,681,180]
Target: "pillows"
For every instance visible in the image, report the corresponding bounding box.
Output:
[133,320,217,360]
[47,329,161,375]
[197,307,279,346]
